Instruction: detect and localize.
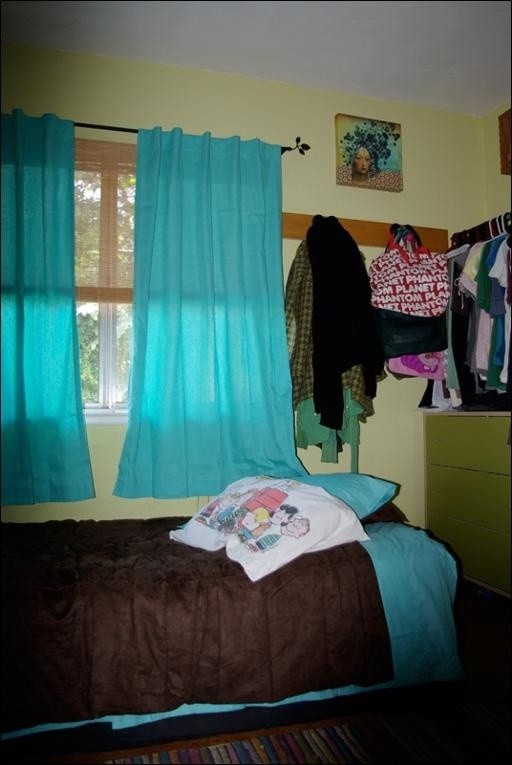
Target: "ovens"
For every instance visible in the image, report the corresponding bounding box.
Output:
[1,515,464,754]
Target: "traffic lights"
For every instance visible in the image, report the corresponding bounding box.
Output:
[294,473,400,520]
[360,503,409,526]
[168,475,369,583]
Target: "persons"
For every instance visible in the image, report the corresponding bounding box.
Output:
[340,120,401,186]
[248,517,311,552]
[238,504,298,543]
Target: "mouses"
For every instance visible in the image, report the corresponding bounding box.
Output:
[457,211,512,248]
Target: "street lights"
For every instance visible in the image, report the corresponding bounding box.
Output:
[368,249,451,381]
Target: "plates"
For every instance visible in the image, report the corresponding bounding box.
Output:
[422,411,510,600]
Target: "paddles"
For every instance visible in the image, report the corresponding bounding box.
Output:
[36,693,512,764]
